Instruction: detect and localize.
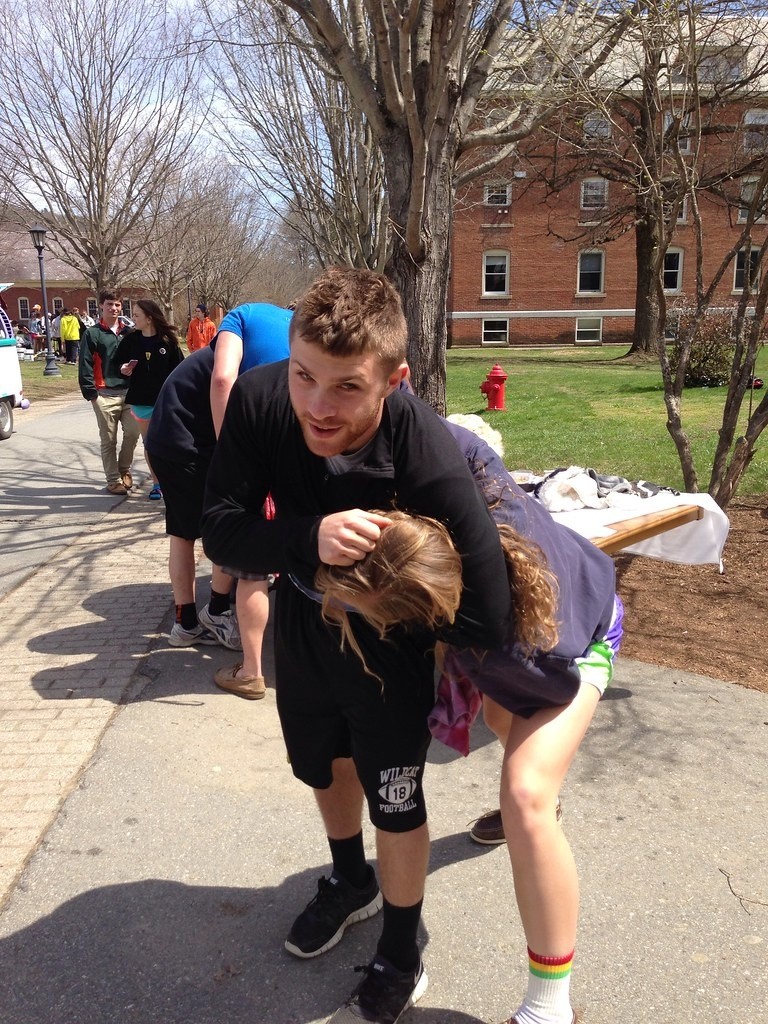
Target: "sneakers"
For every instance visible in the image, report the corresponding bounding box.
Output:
[149,483,161,499]
[196,602,244,653]
[66,361,76,364]
[325,942,428,1023]
[468,792,565,845]
[168,618,223,647]
[285,864,382,958]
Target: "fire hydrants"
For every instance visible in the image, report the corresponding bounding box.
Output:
[478,363,506,411]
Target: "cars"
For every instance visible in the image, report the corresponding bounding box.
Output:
[0,280,28,440]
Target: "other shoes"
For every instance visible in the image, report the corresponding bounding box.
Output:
[214,665,266,700]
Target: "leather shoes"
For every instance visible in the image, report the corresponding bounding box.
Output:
[117,461,132,489]
[106,482,127,495]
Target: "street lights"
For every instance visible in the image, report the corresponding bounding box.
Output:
[184,269,195,330]
[27,221,61,376]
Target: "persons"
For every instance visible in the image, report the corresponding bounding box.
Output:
[209,301,296,701]
[315,359,626,1024]
[144,344,241,650]
[187,304,216,353]
[201,268,514,1024]
[8,304,101,366]
[79,287,141,495]
[109,299,185,499]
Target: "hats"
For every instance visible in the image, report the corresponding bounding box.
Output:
[63,307,72,314]
[197,303,206,311]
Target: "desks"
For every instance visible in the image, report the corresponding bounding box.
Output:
[555,505,705,554]
[34,335,47,354]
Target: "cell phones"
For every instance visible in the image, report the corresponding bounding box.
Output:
[127,360,139,369]
